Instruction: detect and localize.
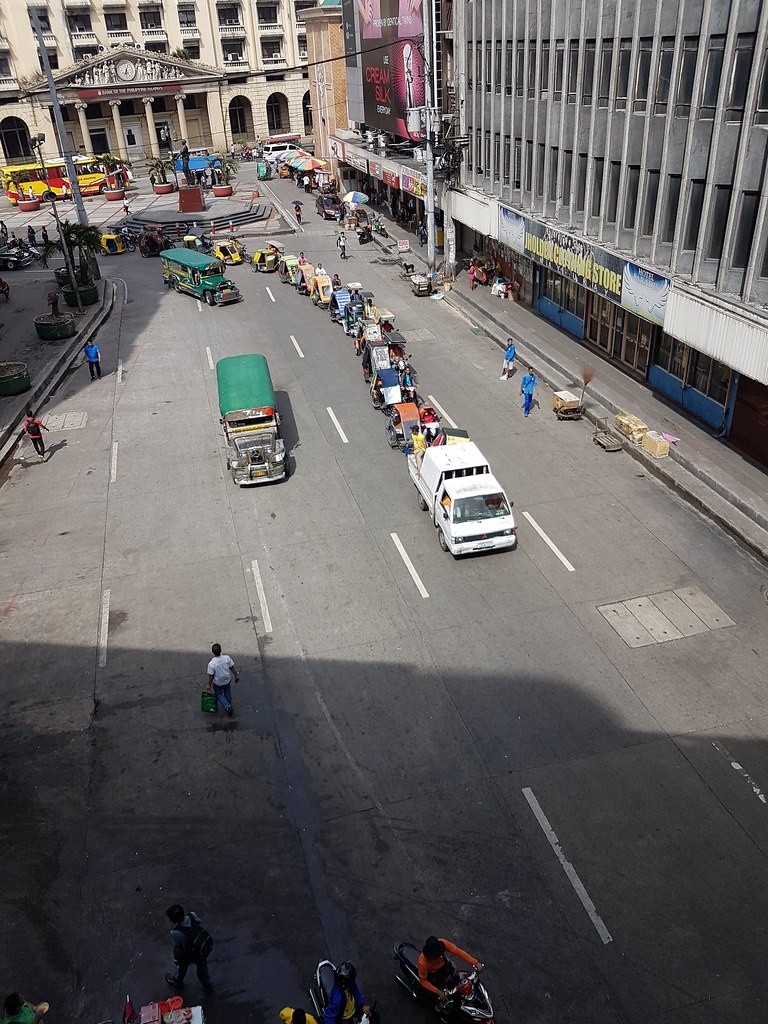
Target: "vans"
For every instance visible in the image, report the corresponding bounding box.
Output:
[167,147,224,175]
[160,247,244,307]
[263,143,305,164]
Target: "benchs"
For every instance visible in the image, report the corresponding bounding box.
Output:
[224,252,232,261]
[258,262,266,269]
[394,420,408,441]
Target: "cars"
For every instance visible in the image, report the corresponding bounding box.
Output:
[316,194,347,220]
[300,143,315,157]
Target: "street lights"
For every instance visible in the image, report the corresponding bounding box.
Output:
[28,133,86,313]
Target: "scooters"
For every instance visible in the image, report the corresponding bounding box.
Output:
[390,939,494,1024]
[356,223,374,245]
[376,222,389,239]
[237,151,253,163]
[308,958,371,1024]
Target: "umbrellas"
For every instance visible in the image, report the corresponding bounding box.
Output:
[288,156,327,171]
[275,150,311,160]
[342,191,369,204]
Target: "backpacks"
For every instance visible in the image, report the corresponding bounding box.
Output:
[25,418,40,436]
[173,913,213,963]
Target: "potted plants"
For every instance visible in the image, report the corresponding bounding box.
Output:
[93,154,133,199]
[144,154,176,194]
[33,209,115,341]
[204,155,240,197]
[4,168,40,211]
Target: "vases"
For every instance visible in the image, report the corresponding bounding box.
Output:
[0,361,31,397]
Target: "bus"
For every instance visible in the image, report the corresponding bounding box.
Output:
[1,153,131,206]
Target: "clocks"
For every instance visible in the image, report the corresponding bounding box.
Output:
[120,63,133,77]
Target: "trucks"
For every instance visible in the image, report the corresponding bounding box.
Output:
[216,353,291,487]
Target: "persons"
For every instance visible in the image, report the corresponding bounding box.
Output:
[83,339,101,382]
[0,220,49,248]
[123,197,131,215]
[269,220,539,475]
[62,183,70,202]
[230,144,249,160]
[196,169,222,189]
[0,906,499,1024]
[83,58,180,84]
[24,410,49,462]
[255,135,357,224]
[0,278,10,303]
[29,186,34,198]
[174,139,195,188]
[207,643,239,717]
[149,174,156,191]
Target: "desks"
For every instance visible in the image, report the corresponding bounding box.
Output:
[508,291,519,302]
[553,391,580,412]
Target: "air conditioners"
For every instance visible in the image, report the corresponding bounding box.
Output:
[301,51,307,56]
[83,54,92,59]
[378,134,386,147]
[272,53,280,58]
[147,23,155,28]
[413,148,443,169]
[41,21,48,29]
[135,42,146,50]
[99,45,105,53]
[365,130,373,143]
[227,19,239,24]
[228,53,239,61]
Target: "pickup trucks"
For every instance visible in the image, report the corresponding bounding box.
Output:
[407,442,518,556]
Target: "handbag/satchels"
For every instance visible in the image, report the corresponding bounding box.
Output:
[201,688,218,713]
[123,208,125,212]
[522,389,525,394]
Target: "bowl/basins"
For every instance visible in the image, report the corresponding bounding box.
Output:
[163,996,192,1024]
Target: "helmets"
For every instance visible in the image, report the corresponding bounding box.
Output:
[333,962,357,989]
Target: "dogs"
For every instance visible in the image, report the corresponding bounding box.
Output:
[403,263,414,272]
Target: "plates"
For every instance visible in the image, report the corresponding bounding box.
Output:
[36,1001,49,1015]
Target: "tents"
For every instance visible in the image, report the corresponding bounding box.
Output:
[175,156,221,170]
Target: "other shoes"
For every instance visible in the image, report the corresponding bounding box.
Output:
[165,973,184,989]
[228,707,233,717]
[38,455,46,462]
[344,259,347,261]
[202,982,215,996]
[339,255,342,259]
[91,376,96,381]
[525,414,528,417]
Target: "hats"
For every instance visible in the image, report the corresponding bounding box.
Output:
[423,402,433,408]
[423,936,445,958]
[293,1008,306,1024]
[410,425,419,431]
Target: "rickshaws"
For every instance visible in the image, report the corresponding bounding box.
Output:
[0,233,37,271]
[182,226,253,266]
[136,224,176,258]
[99,224,139,257]
[251,240,287,274]
[385,402,447,453]
[368,364,421,417]
[275,255,414,385]
